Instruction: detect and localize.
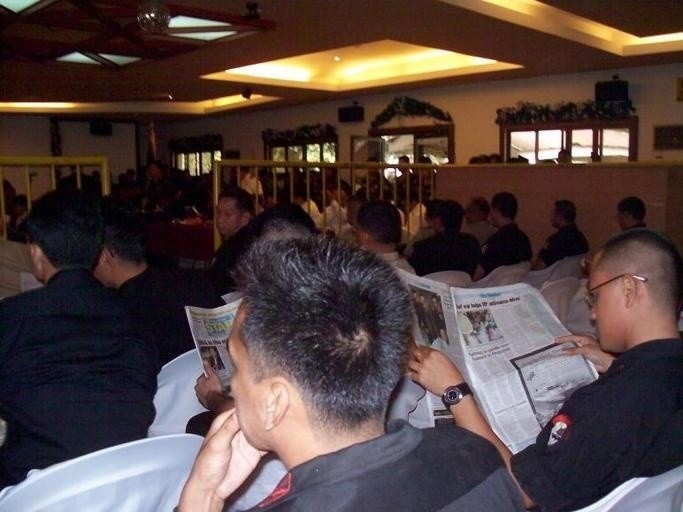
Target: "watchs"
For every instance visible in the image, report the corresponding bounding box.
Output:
[440,380,474,412]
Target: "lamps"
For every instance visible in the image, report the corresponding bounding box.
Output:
[136,0,170,32]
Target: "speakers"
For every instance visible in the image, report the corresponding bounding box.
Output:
[338,106,364,122]
[89,120,112,137]
[595,80,628,101]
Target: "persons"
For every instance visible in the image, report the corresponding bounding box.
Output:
[1,184,163,486]
[175,231,528,511]
[406,224,683,511]
[184,360,239,439]
[607,193,653,248]
[534,198,601,271]
[1,145,531,363]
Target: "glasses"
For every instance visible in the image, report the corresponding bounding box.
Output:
[584,274,647,307]
